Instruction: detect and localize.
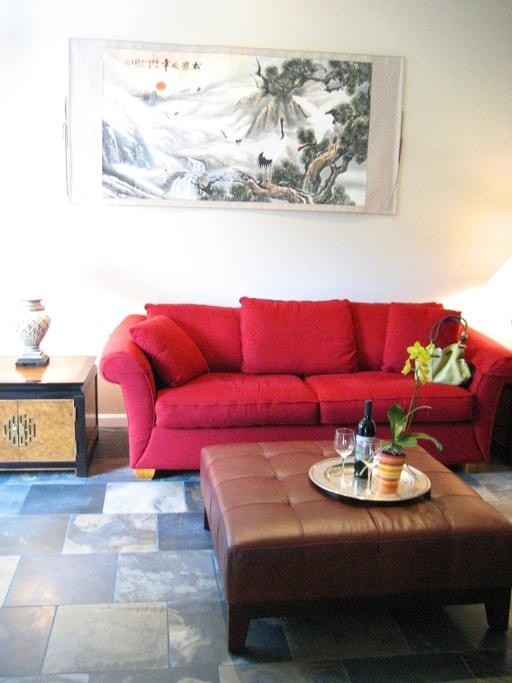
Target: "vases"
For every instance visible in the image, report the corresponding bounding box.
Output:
[379,454,404,487]
[14,297,51,366]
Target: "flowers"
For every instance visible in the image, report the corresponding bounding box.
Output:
[383,339,443,456]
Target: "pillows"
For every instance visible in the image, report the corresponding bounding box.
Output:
[351,302,444,370]
[381,302,462,373]
[130,315,209,388]
[144,304,242,371]
[238,299,359,374]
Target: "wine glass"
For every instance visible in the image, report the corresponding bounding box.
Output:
[332,425,356,479]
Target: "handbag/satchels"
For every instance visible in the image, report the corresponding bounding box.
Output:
[415,315,472,386]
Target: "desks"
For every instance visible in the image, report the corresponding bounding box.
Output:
[1,355,100,476]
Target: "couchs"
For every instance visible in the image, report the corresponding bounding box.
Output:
[99,313,512,479]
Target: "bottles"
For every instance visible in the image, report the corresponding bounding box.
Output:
[353,397,377,481]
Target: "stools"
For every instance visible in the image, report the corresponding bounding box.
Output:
[201,439,512,657]
